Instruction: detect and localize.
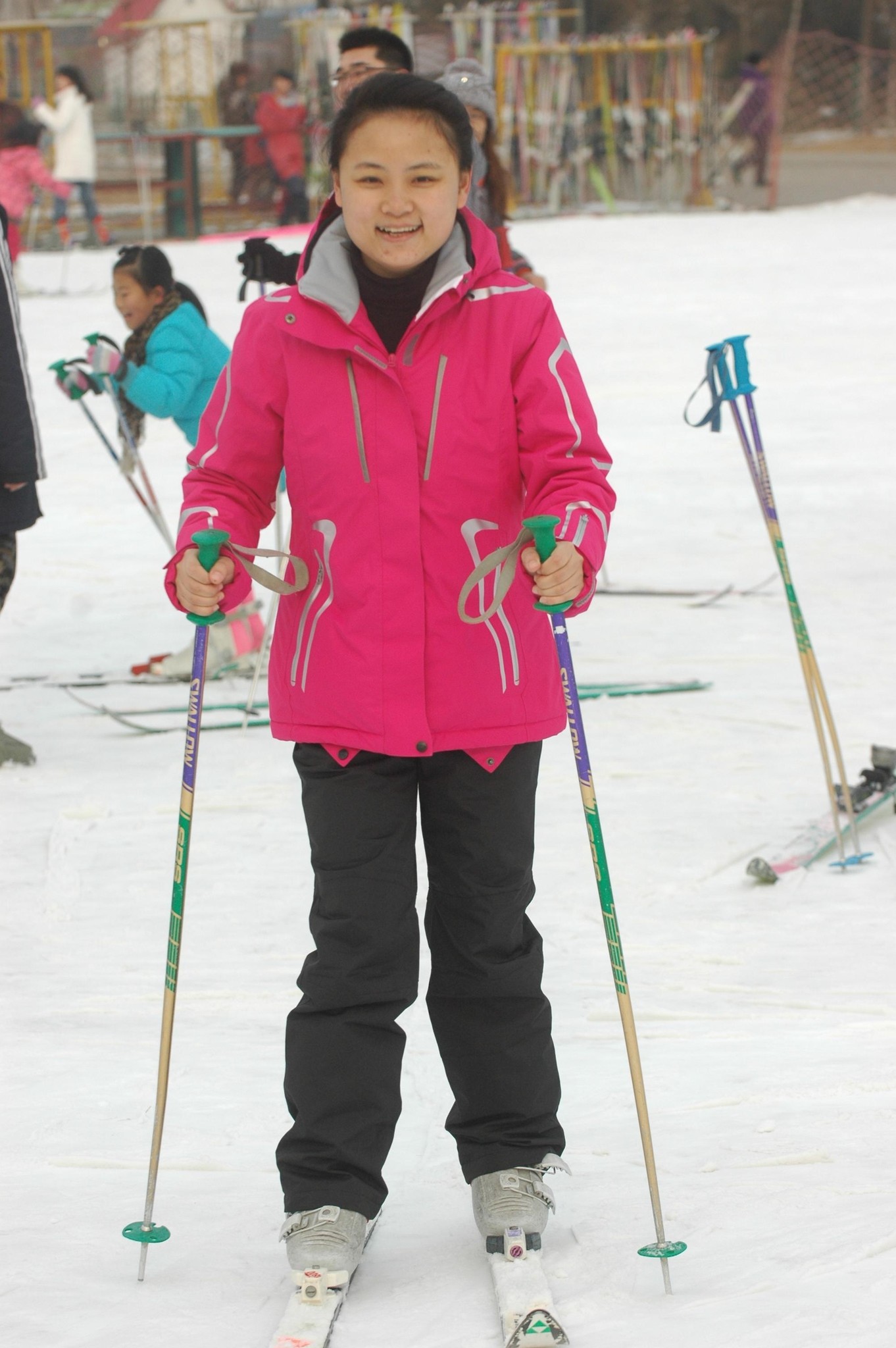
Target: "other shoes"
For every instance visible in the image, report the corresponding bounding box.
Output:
[157,634,242,675]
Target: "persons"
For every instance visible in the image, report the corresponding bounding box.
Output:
[0,100,81,263]
[216,60,258,200]
[332,26,414,109]
[729,50,774,187]
[254,68,310,225]
[433,58,514,228]
[164,73,616,1277]
[55,246,286,676]
[0,203,46,612]
[32,63,111,251]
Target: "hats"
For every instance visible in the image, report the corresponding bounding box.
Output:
[431,58,495,132]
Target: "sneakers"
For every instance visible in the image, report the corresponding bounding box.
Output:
[470,1163,551,1237]
[285,1206,367,1275]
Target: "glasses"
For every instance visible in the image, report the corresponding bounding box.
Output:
[327,66,399,86]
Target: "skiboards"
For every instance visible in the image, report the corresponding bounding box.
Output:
[742,738,896,885]
[265,1167,572,1348]
[583,572,780,616]
[1,663,713,738]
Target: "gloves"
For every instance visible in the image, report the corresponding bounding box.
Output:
[87,344,120,375]
[238,243,286,285]
[55,371,89,400]
